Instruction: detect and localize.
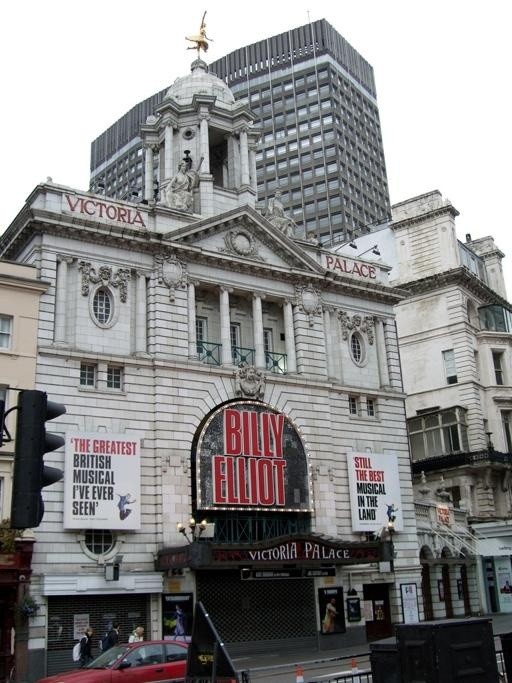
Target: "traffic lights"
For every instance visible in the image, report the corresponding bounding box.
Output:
[10,389,67,529]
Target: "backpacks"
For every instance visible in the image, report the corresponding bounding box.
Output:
[73,635,89,663]
[98,630,119,651]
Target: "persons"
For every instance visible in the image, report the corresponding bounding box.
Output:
[107,623,121,647]
[156,159,195,211]
[239,365,261,396]
[128,626,144,643]
[79,627,96,667]
[117,492,137,520]
[321,598,338,633]
[385,502,399,522]
[266,191,298,234]
[170,604,188,642]
[307,231,319,244]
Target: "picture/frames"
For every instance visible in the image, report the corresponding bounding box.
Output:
[162,593,193,636]
[438,580,445,601]
[318,586,346,635]
[400,583,421,624]
[457,580,464,600]
[347,598,361,622]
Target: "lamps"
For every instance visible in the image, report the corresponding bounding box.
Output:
[387,521,396,542]
[177,518,208,545]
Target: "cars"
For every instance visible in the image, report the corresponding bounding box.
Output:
[35,638,189,683]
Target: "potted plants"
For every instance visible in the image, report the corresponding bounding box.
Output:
[13,593,41,617]
